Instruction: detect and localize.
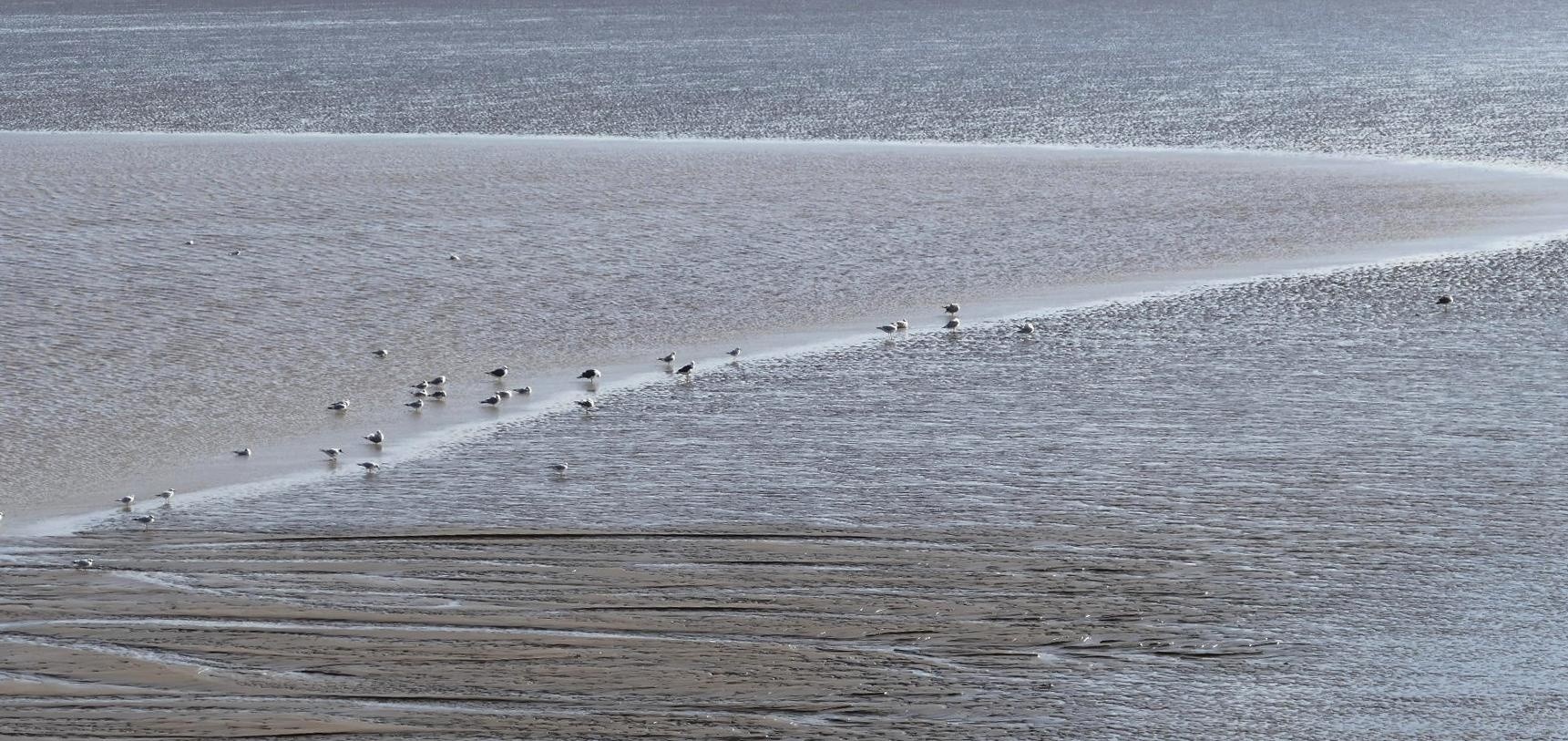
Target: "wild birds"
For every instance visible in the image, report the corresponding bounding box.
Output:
[185,241,240,256]
[364,430,383,444]
[942,303,959,331]
[1015,324,1034,336]
[320,447,343,458]
[1435,294,1454,308]
[479,367,532,405]
[114,487,176,531]
[357,463,379,473]
[404,375,447,413]
[657,351,694,380]
[545,463,568,476]
[726,348,740,358]
[327,400,349,410]
[232,449,250,456]
[574,369,601,411]
[371,350,387,357]
[876,318,907,336]
[447,255,459,260]
[71,558,93,569]
